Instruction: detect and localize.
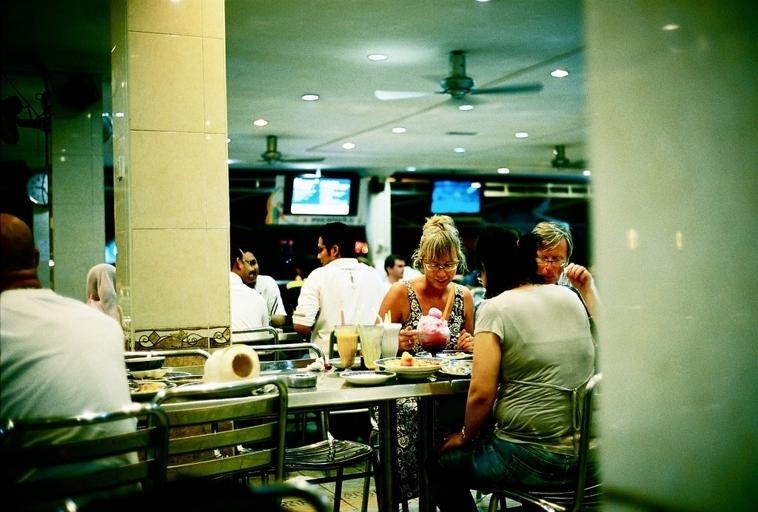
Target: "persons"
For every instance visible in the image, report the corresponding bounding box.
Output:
[374,214,599,512]
[0,210,147,512]
[229,222,424,446]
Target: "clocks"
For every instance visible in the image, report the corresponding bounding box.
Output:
[25,169,50,207]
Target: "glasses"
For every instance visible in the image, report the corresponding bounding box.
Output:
[537,256,566,266]
[422,261,458,271]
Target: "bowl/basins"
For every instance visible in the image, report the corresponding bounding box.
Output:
[124,357,167,371]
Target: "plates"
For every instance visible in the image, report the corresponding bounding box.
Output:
[339,357,473,385]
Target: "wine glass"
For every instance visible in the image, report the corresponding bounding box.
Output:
[334,323,402,375]
[417,308,451,357]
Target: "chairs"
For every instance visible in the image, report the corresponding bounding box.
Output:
[145,376,287,487]
[193,479,334,511]
[1,404,168,512]
[237,343,375,512]
[230,326,281,361]
[487,371,602,510]
[122,349,212,375]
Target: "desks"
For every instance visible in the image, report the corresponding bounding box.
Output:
[270,330,301,342]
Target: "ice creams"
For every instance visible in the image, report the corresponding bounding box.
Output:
[418,307,451,347]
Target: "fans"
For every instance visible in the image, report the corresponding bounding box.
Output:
[423,53,544,108]
[545,146,587,171]
[246,137,325,164]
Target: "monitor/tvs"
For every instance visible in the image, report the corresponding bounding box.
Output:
[430,180,484,216]
[284,172,360,216]
